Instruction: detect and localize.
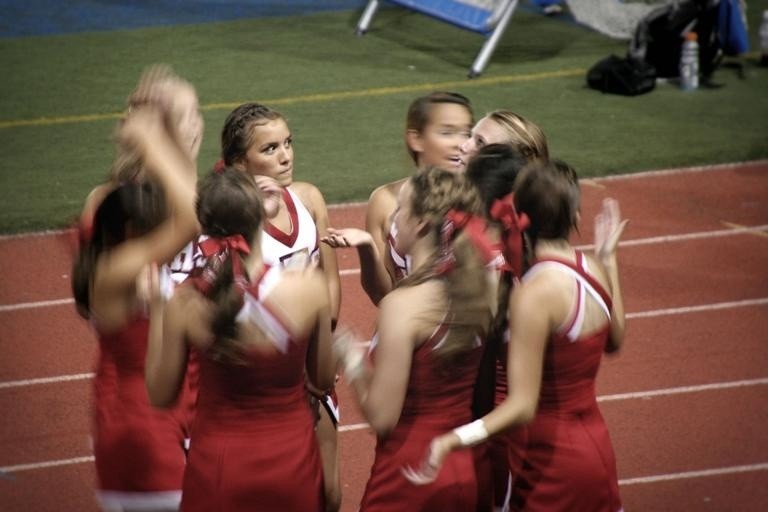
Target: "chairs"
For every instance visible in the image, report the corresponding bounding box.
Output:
[352,0,750,76]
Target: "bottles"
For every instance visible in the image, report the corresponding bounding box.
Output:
[679,31,701,93]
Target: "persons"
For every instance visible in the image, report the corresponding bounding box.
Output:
[71,66,628,509]
